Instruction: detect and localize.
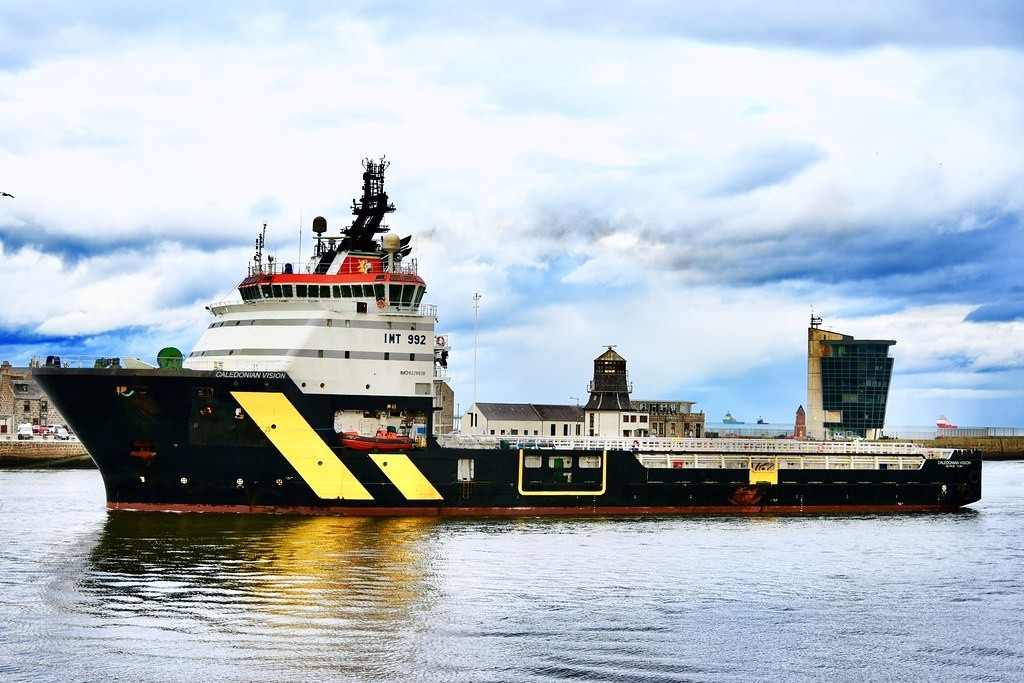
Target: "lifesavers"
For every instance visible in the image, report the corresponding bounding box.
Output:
[929,451,934,457]
[377,300,385,308]
[818,444,824,452]
[632,440,639,451]
[436,337,444,345]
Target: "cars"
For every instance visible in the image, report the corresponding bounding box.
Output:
[47,423,62,433]
[28,159,984,517]
[33,424,49,434]
